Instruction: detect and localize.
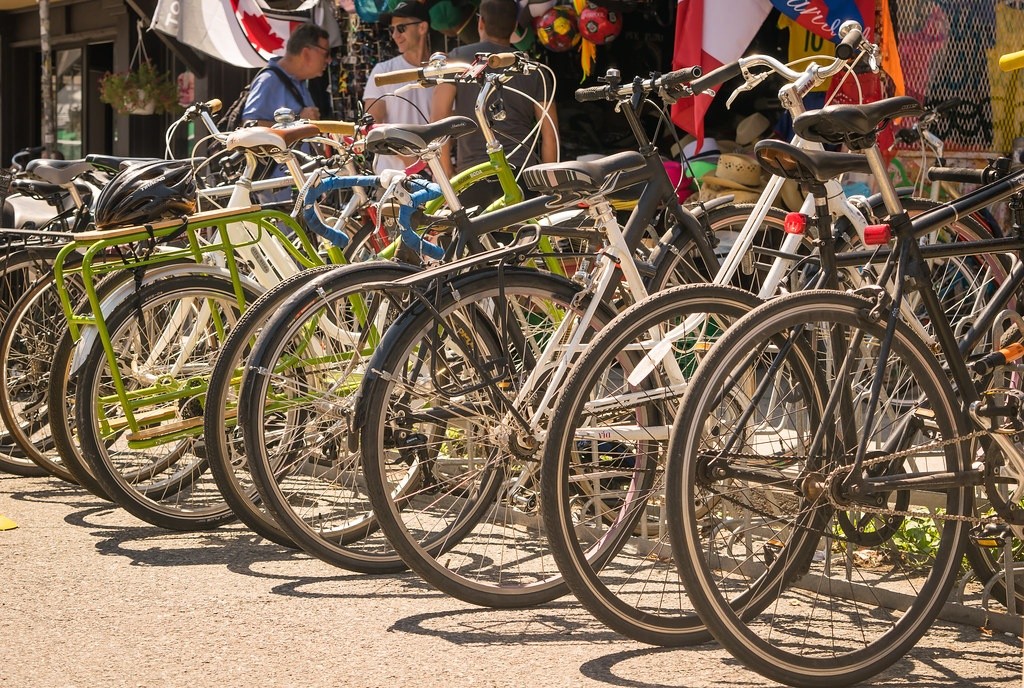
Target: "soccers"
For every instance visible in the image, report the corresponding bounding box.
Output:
[579,3,622,44]
[537,5,581,52]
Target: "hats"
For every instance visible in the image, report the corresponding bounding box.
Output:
[669,112,810,268]
[388,0,430,24]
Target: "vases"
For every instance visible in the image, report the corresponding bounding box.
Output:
[122,89,154,113]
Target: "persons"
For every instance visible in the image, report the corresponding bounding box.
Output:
[427,0,563,247]
[243,26,334,271]
[360,5,454,242]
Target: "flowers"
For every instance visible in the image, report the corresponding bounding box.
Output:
[99,61,182,114]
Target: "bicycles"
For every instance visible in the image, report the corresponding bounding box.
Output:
[2,51,1023,688]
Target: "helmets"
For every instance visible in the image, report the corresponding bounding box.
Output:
[93,159,197,230]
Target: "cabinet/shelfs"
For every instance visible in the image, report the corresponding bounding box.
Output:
[326,16,404,124]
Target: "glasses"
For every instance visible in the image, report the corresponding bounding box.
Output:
[388,21,422,34]
[306,42,331,59]
